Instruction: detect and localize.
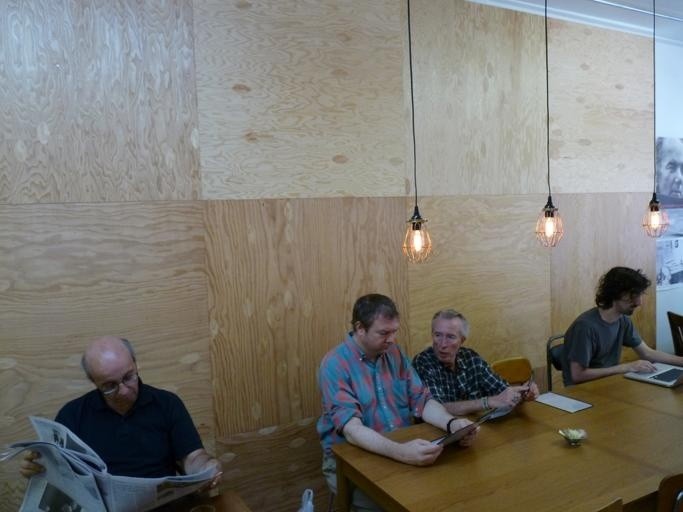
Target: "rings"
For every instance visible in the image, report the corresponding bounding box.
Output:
[212,481,217,486]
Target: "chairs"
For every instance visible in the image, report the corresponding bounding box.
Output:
[489,355,535,387]
[663,307,683,356]
[592,496,625,511]
[654,469,682,511]
[544,326,571,395]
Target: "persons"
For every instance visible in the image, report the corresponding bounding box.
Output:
[409,308,539,416]
[18,334,223,511]
[315,292,481,512]
[561,266,683,385]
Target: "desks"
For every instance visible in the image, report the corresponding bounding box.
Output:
[331,355,682,512]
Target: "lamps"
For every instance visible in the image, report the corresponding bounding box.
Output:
[527,1,569,255]
[400,1,435,270]
[638,3,671,242]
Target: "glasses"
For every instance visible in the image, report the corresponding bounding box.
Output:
[100,371,138,395]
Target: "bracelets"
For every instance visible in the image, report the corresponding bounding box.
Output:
[445,417,458,434]
[480,395,489,410]
